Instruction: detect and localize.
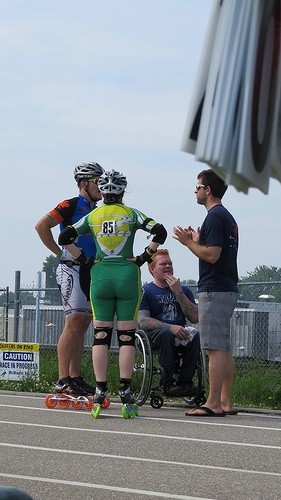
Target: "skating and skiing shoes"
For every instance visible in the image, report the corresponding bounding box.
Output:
[44,375,110,410]
[118,378,139,419]
[91,380,109,418]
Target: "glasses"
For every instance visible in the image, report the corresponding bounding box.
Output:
[88,179,99,184]
[196,184,205,190]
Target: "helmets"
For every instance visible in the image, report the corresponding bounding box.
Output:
[97,169,127,194]
[73,162,105,182]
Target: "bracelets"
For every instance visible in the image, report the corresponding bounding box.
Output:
[147,246,157,254]
[57,250,63,258]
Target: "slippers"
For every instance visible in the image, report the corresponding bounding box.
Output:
[185,404,226,417]
[225,409,239,415]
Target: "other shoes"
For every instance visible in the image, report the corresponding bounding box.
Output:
[160,375,198,397]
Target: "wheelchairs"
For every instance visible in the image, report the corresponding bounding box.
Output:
[127,331,209,410]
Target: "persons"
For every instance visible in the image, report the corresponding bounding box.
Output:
[58,169,167,405]
[172,169,239,417]
[136,249,200,398]
[34,161,111,399]
